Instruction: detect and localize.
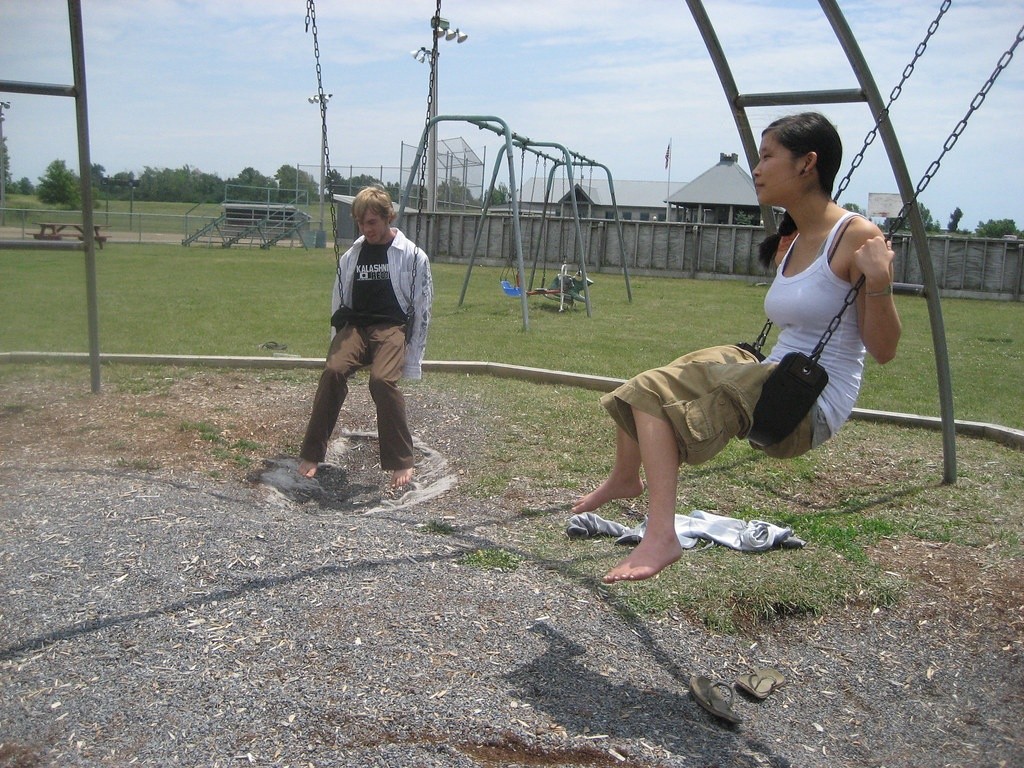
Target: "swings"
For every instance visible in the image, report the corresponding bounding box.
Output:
[305,1,444,357]
[730,0,1024,445]
[501,147,595,306]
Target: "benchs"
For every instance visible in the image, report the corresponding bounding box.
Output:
[26,233,113,250]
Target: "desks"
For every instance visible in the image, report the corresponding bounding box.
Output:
[33,222,112,235]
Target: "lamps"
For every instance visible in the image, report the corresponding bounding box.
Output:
[652,216,658,221]
[760,220,765,226]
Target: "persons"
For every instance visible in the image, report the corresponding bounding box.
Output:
[298,183,434,488]
[571,113,902,583]
